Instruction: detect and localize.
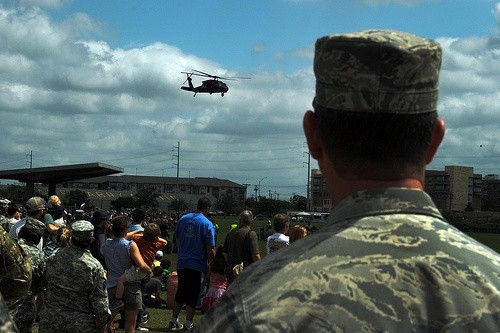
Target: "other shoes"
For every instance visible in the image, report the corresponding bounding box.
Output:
[169,321,184,330]
[137,326,149,332]
[183,323,195,333]
[111,300,124,310]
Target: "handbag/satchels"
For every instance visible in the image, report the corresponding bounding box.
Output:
[232,229,255,279]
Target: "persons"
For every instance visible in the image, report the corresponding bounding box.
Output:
[169,196,215,331]
[211,218,219,247]
[128,209,145,231]
[110,223,168,311]
[38,220,112,333]
[143,251,171,309]
[230,222,238,231]
[0,195,85,295]
[266,213,290,254]
[100,217,152,333]
[192,29,500,333]
[223,210,261,283]
[0,226,36,333]
[266,216,272,232]
[141,210,187,254]
[202,246,227,313]
[90,209,108,270]
[289,225,307,243]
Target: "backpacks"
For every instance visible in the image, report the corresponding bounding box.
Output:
[136,303,150,326]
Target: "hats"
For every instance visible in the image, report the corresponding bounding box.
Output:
[71,221,94,241]
[314,29,442,114]
[24,217,47,236]
[25,196,52,211]
[0,200,9,208]
[90,210,110,220]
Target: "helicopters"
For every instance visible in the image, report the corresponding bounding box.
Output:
[180,68,253,97]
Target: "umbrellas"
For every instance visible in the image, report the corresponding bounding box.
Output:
[296,212,311,217]
